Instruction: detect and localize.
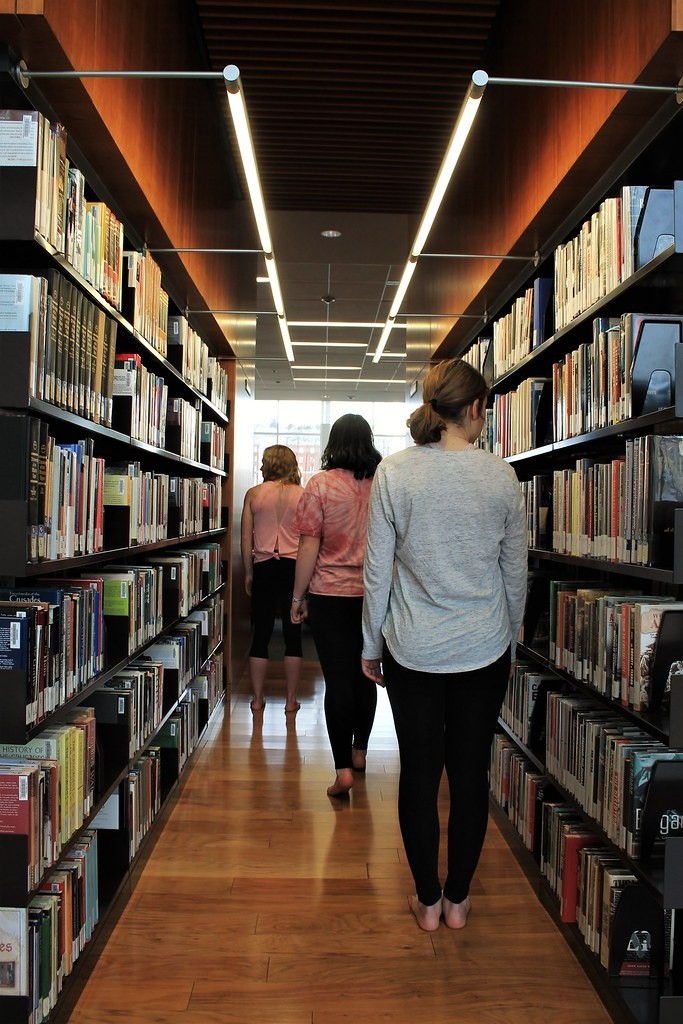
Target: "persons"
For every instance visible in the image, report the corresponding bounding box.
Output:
[290,414,383,796]
[362,358,530,932]
[241,445,304,712]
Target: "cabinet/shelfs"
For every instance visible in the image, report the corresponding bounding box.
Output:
[456,94,683,1024]
[0,46,237,1024]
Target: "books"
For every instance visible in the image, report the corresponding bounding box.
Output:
[0,110,683,1023]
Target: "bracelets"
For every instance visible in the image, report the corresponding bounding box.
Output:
[293,597,305,603]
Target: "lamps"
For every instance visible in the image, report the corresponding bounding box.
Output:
[370,69,683,367]
[16,56,296,365]
